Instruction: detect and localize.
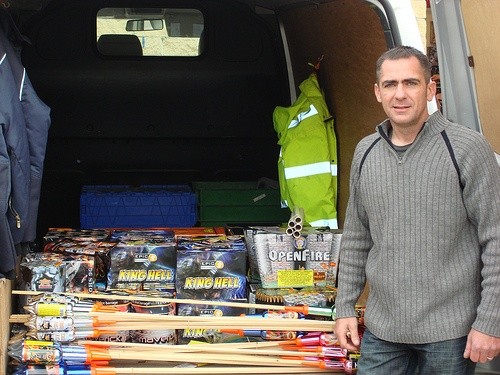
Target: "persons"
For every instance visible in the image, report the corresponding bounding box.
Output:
[336,46,500,375]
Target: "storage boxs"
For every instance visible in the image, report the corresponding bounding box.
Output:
[0,226,343,375]
[79,181,292,229]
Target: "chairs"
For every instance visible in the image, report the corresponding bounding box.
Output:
[97,34,142,56]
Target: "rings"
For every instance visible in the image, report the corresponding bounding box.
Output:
[487,357,493,360]
